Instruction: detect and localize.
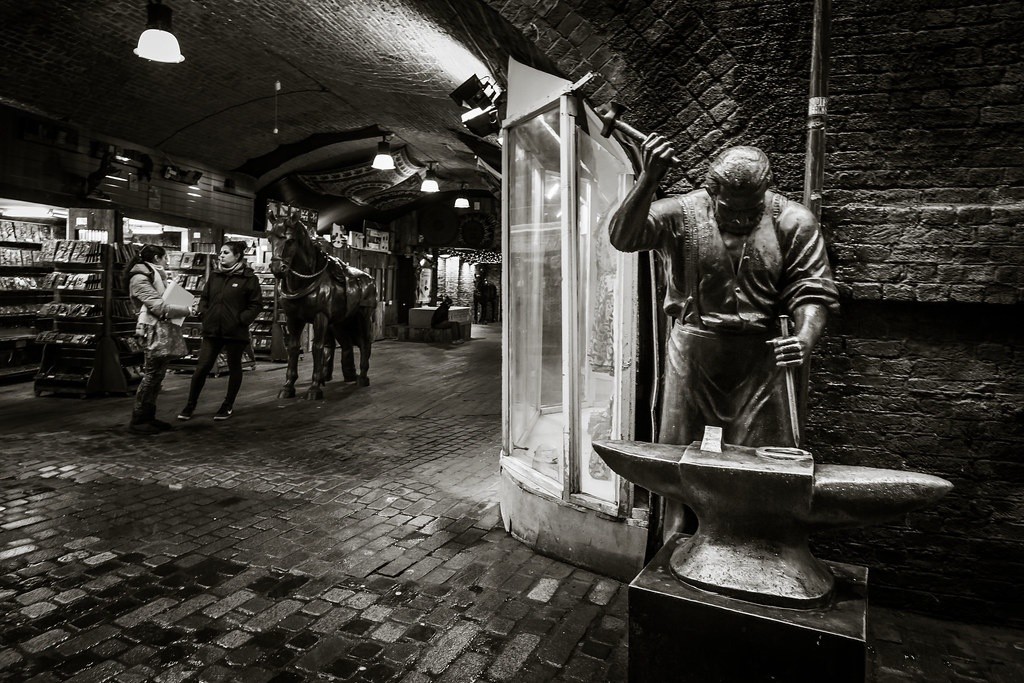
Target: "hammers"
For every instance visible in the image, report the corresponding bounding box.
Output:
[601,101,682,167]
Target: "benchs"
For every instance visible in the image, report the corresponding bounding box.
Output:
[459,321,471,340]
[391,325,447,344]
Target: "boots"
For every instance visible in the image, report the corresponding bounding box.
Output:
[129,406,172,435]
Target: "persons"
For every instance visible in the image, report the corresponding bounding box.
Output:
[608,133,842,455]
[177,240,264,421]
[430,297,466,344]
[126,243,192,439]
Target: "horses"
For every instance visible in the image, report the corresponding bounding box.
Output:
[267,208,377,401]
[473,272,498,324]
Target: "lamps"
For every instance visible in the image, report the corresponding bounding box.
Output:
[372,134,397,171]
[460,104,499,138]
[448,73,497,111]
[454,182,470,209]
[420,163,440,193]
[132,3,186,64]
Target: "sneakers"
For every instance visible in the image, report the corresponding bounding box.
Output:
[214,406,233,420]
[178,406,194,420]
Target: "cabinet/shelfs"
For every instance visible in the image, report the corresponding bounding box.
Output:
[0,214,302,398]
